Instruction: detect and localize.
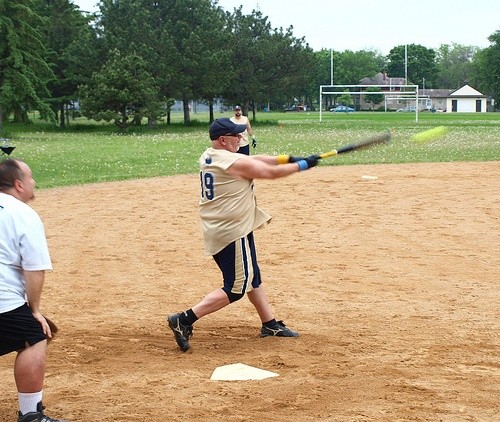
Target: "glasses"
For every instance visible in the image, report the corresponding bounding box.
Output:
[222,133,239,137]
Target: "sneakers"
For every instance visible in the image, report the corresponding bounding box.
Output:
[166,313,193,352]
[262,321,299,337]
[16,401,63,422]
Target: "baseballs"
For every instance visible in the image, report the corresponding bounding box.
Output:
[435,126,446,136]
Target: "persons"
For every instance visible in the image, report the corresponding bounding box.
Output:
[229,104,257,201]
[169,117,387,351]
[0,160,61,422]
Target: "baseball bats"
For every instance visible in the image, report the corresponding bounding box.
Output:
[319,134,392,158]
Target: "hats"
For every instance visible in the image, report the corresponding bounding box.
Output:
[234,106,241,111]
[209,118,246,140]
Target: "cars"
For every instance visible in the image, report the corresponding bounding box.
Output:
[330,105,356,112]
[284,103,308,111]
[396,105,416,112]
